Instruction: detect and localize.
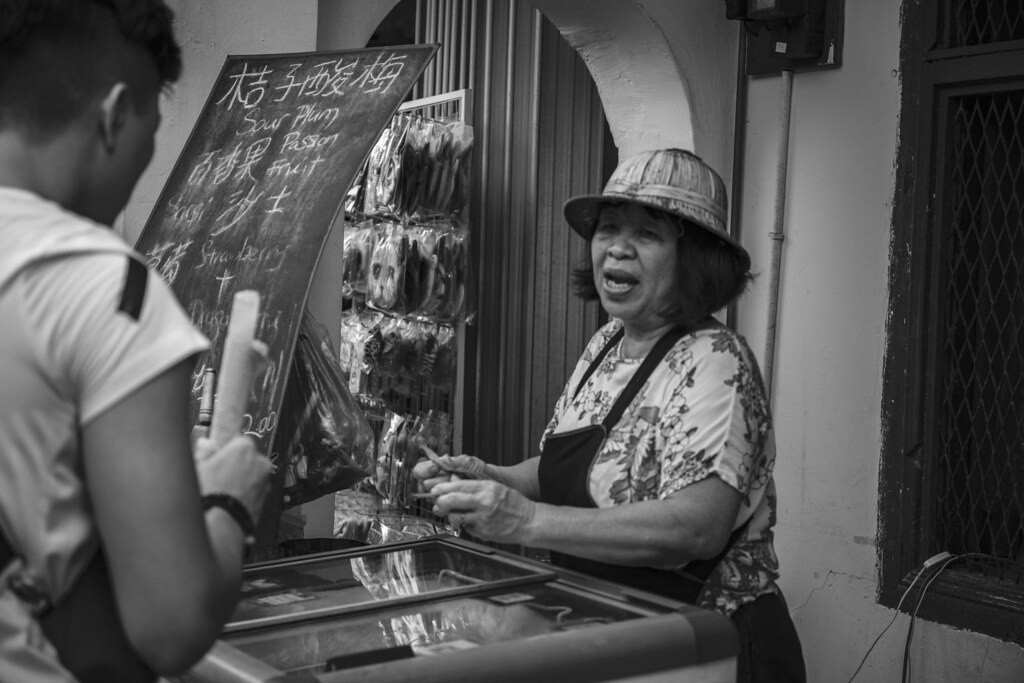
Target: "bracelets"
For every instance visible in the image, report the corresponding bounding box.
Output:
[200,491,254,535]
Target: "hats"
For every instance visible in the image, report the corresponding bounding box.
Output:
[564,148,752,274]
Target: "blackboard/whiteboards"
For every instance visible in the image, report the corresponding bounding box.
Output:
[132,40,442,564]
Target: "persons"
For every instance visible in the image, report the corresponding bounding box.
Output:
[1,1,277,682]
[406,149,810,682]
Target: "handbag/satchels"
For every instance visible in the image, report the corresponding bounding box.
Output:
[0,536,157,680]
